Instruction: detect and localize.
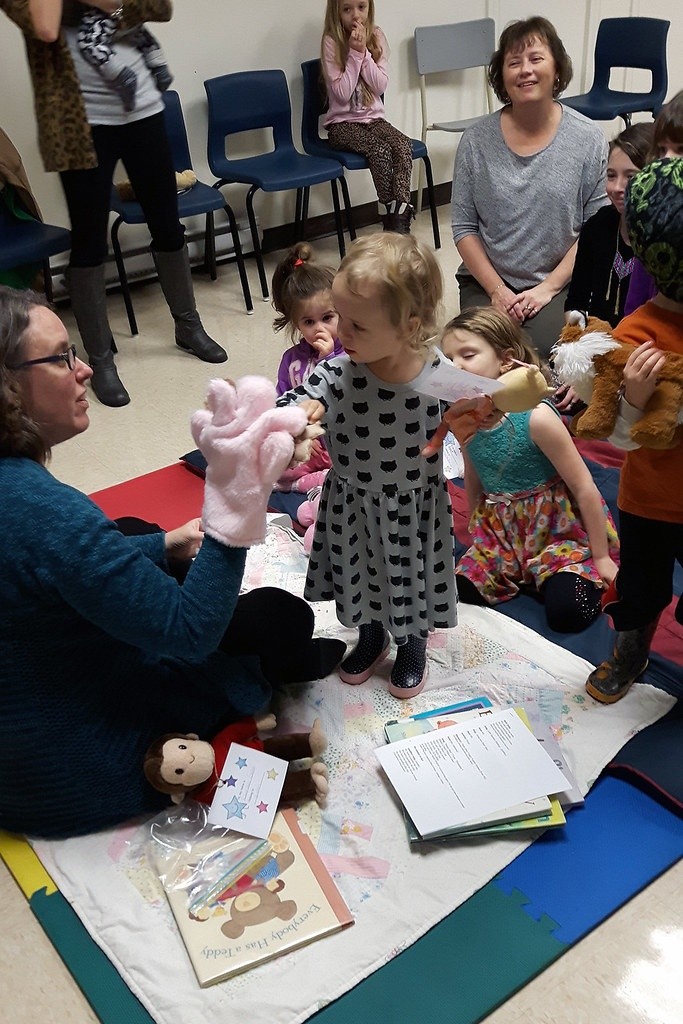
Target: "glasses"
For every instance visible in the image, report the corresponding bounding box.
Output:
[8,343,76,372]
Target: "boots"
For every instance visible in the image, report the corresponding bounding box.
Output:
[65,264,130,406]
[149,237,230,364]
[388,630,430,700]
[585,613,661,704]
[378,200,416,234]
[339,620,391,685]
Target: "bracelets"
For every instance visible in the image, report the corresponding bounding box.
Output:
[490,283,506,298]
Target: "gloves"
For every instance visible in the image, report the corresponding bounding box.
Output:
[189,375,310,550]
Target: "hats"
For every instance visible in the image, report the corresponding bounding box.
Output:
[624,157,682,302]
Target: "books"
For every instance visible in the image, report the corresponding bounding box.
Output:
[146,798,356,988]
[370,690,587,844]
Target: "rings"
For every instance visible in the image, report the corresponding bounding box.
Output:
[563,383,567,388]
[570,400,575,406]
[533,309,539,313]
[528,305,532,310]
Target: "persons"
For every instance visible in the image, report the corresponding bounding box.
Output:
[0,285,347,839]
[60,0,173,112]
[556,90,683,704]
[316,0,416,235]
[441,304,620,634]
[294,233,495,699]
[0,0,230,407]
[450,15,612,369]
[268,245,333,494]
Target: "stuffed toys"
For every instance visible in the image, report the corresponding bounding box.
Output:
[549,308,683,450]
[190,375,308,547]
[142,710,328,811]
[297,492,326,553]
[422,363,557,457]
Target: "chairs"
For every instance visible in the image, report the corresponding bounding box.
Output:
[205,69,357,302]
[1,186,78,295]
[301,57,443,258]
[415,19,494,215]
[558,16,671,129]
[111,91,254,338]
